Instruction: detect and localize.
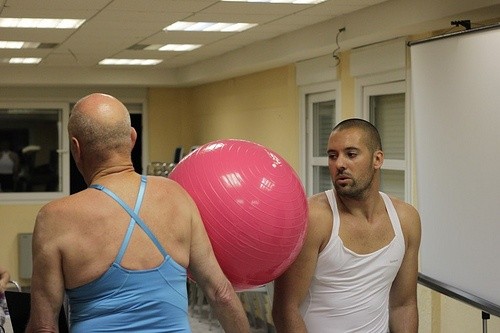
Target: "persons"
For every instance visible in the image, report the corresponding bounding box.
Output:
[25,93,251,333]
[0,267,14,333]
[271,118,422,333]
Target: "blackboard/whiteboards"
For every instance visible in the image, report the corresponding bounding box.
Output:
[404,25,500,318]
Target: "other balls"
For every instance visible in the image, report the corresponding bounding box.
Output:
[167,138,310,294]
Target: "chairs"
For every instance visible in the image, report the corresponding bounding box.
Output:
[4,290,69,333]
[190,286,269,333]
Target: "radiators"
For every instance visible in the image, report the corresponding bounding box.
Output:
[17,233,34,279]
